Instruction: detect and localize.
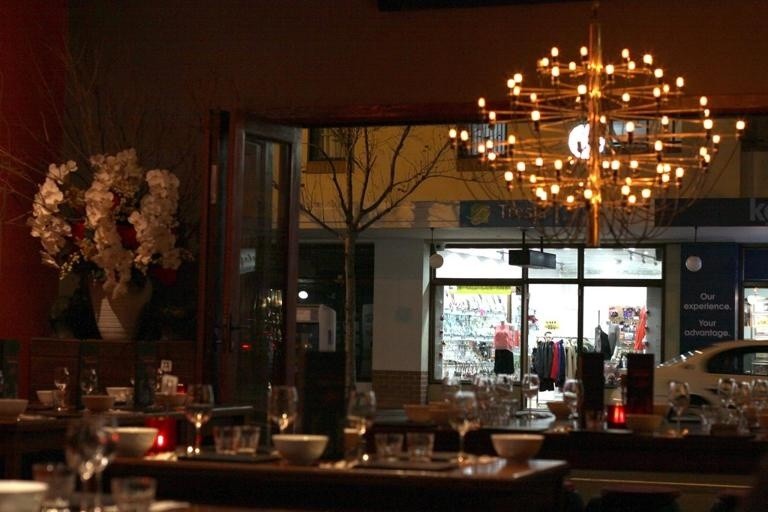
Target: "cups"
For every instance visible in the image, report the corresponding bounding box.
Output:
[234,426,259,453]
[407,432,431,463]
[213,425,236,454]
[32,464,73,512]
[376,431,402,462]
[113,477,154,511]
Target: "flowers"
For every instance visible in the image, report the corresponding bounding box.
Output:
[28,148,202,299]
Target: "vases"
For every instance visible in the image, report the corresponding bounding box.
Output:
[89,271,154,342]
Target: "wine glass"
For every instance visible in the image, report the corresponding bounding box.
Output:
[751,379,766,426]
[64,418,104,512]
[346,390,376,468]
[496,374,514,418]
[270,385,297,449]
[563,378,582,428]
[478,378,496,418]
[185,385,216,455]
[521,374,538,411]
[717,378,735,423]
[734,382,750,427]
[668,381,690,431]
[446,394,478,464]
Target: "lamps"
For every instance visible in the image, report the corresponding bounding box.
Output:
[568,121,605,161]
[429,227,444,268]
[686,225,702,272]
[448,23,746,247]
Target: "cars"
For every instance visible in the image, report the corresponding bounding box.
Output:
[602,340,768,442]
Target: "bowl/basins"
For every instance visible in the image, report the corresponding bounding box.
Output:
[38,390,65,407]
[547,402,570,419]
[1,399,29,423]
[270,434,328,465]
[1,479,47,512]
[84,394,115,412]
[106,427,156,456]
[490,433,543,465]
[108,387,135,406]
[702,404,736,428]
[404,404,435,423]
